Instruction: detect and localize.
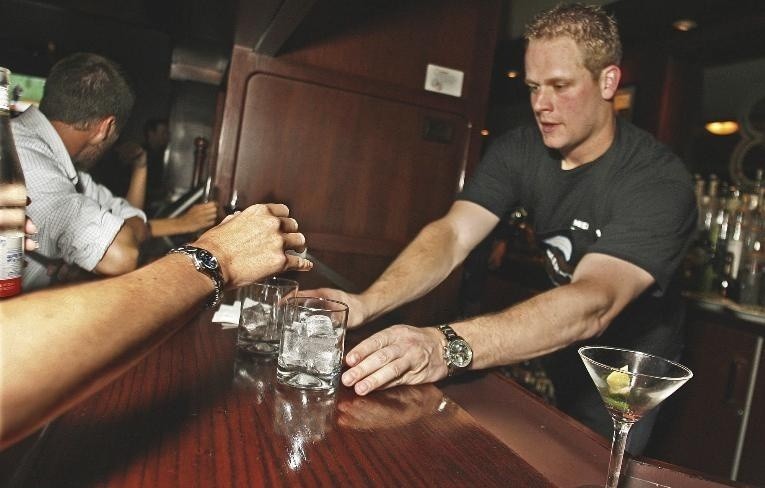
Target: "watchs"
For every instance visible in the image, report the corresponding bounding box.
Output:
[434,322,475,379]
[165,242,225,307]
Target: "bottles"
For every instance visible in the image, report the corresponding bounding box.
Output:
[685,170,765,308]
[0,67,29,300]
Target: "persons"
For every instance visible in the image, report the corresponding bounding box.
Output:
[110,138,222,238]
[140,116,169,199]
[0,193,41,271]
[270,0,701,456]
[6,50,148,289]
[0,201,316,454]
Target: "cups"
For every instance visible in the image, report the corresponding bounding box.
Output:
[271,383,340,444]
[277,293,350,392]
[237,274,301,359]
[230,357,289,400]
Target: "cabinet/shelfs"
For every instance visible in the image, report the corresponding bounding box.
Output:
[665,324,765,480]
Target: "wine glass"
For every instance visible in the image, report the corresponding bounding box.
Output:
[576,343,694,487]
